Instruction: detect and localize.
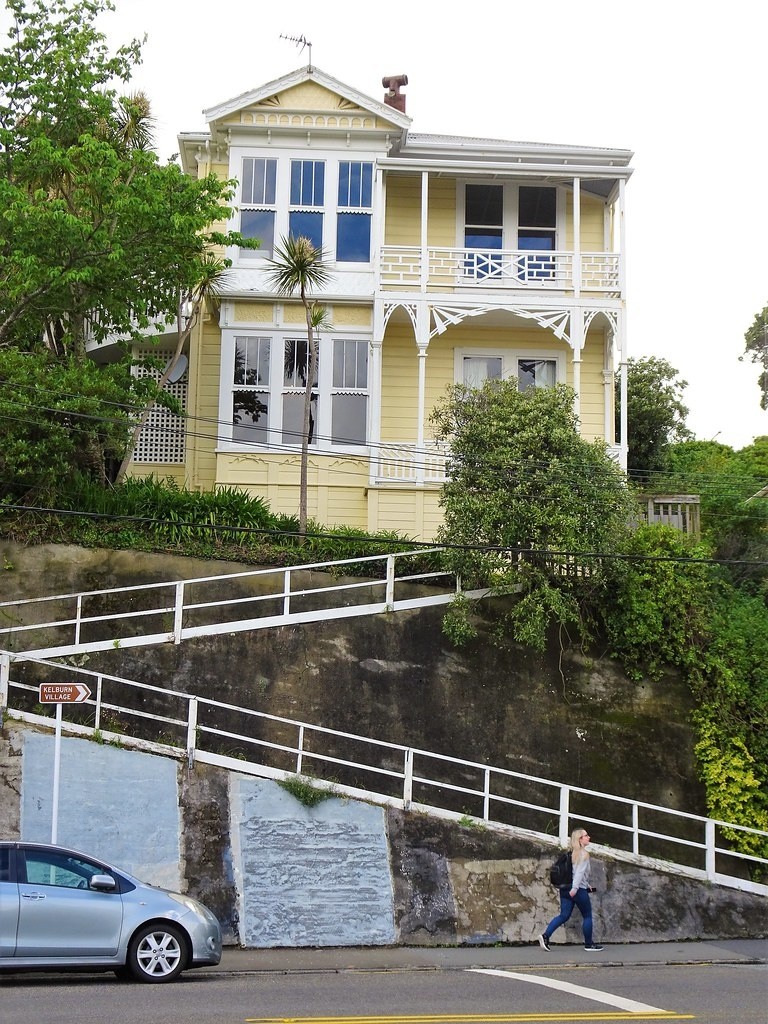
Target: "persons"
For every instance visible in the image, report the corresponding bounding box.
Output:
[539,828,604,951]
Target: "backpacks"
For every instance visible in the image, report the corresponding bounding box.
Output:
[550,850,573,889]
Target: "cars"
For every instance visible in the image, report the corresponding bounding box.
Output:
[0,840,224,980]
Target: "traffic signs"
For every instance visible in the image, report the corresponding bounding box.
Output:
[39,681,92,705]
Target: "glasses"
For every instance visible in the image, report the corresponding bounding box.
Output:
[581,834,589,837]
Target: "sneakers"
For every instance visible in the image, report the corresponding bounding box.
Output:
[537,933,551,952]
[584,945,604,952]
